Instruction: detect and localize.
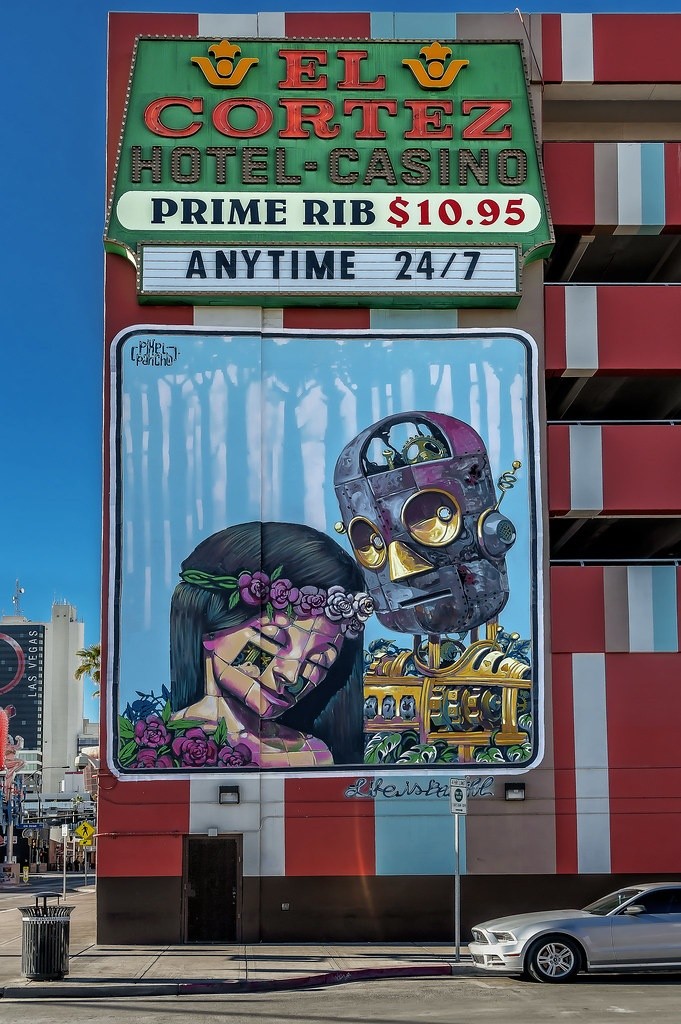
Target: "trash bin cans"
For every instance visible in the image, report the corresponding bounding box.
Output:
[16,891,75,980]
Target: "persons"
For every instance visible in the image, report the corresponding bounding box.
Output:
[67,858,85,872]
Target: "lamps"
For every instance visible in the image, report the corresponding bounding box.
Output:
[505,783,526,801]
[218,785,240,805]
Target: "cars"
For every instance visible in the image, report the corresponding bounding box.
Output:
[467,881,681,983]
